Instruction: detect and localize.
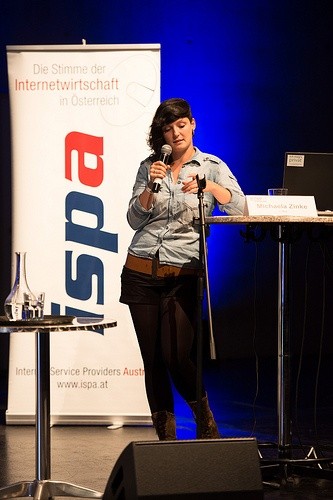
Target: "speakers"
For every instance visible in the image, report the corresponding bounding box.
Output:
[102,438,265,500]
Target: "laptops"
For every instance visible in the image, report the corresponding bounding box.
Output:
[283,151,333,212]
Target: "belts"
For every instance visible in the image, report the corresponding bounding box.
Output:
[124,253,206,277]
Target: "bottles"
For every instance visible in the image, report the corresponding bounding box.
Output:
[4,251,38,321]
[21,293,44,321]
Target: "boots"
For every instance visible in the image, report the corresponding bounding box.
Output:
[152,411,176,441]
[189,391,221,439]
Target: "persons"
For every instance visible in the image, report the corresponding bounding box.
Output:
[119,100,248,440]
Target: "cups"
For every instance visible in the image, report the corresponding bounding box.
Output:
[268,189,288,196]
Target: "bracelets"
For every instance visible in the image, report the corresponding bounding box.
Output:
[146,187,153,195]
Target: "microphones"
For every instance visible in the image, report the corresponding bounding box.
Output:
[152,144,173,193]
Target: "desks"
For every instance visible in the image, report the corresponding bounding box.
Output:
[0,317,117,500]
[205,215,333,470]
